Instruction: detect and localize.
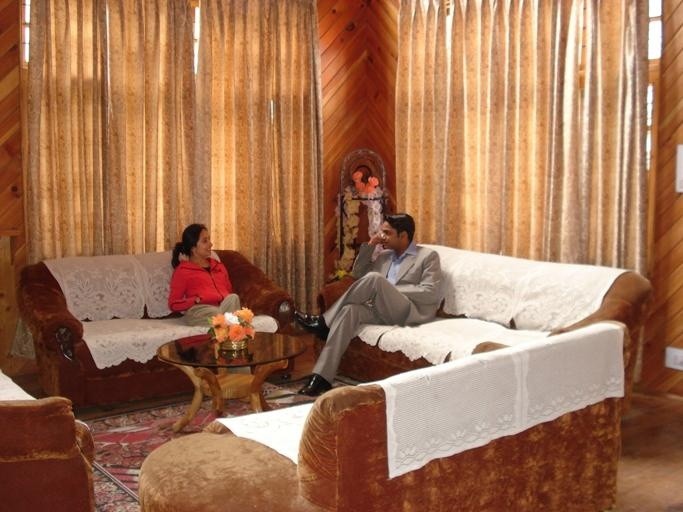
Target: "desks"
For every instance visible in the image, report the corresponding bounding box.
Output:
[157,331,307,431]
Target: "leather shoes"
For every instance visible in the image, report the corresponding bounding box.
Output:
[298,374,333,397]
[294,310,330,341]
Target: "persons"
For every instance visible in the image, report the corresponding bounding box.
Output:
[167,223,251,403]
[293,213,444,397]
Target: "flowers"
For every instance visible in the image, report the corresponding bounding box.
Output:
[207,309,279,355]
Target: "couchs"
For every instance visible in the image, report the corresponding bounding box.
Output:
[1,371,97,512]
[18,251,297,410]
[138,320,631,511]
[313,244,653,414]
